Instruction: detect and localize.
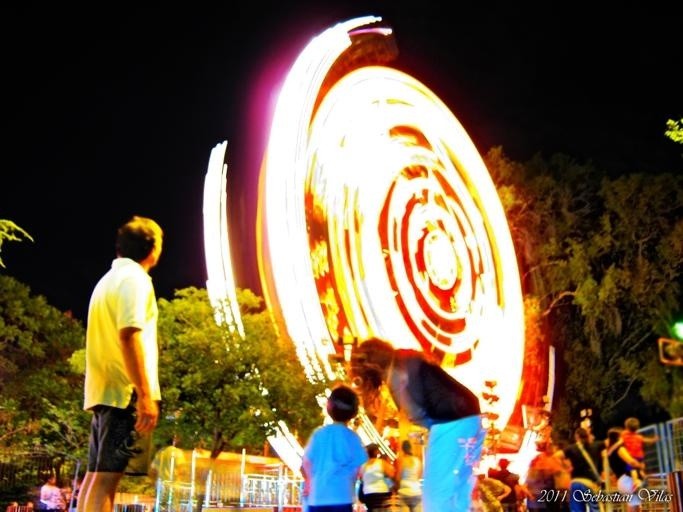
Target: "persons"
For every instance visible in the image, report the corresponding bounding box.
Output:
[298,385,366,511]
[38,474,66,511]
[605,416,658,495]
[76,215,168,512]
[350,337,484,511]
[550,428,609,512]
[606,425,646,512]
[358,439,573,511]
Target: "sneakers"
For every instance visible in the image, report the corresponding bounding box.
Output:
[632,479,642,493]
